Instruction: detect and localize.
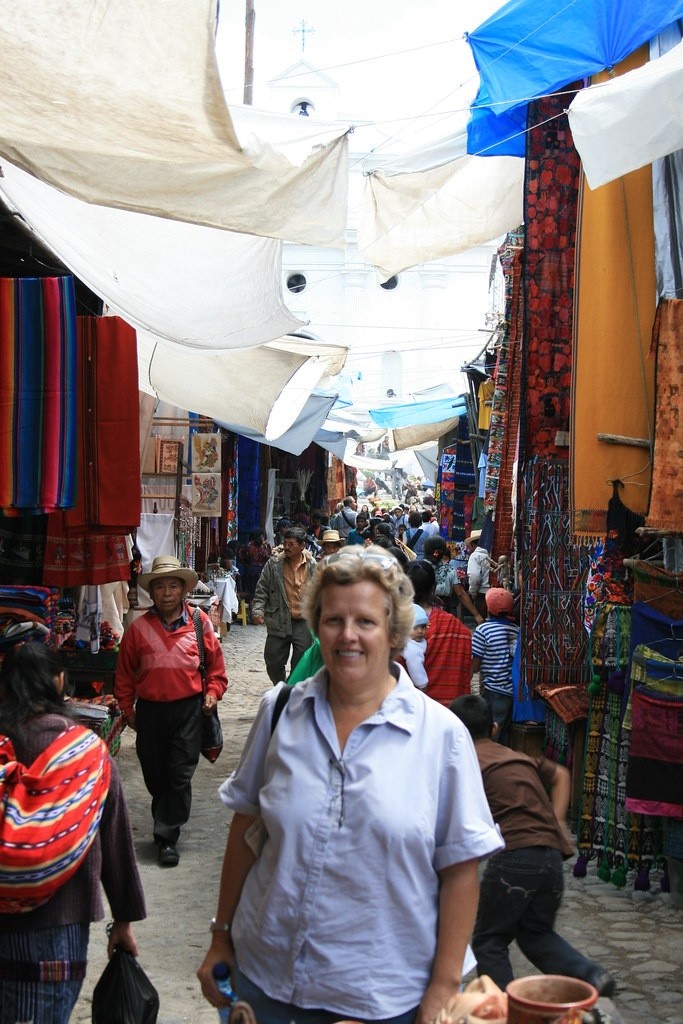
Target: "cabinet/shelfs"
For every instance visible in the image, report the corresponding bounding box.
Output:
[140,441,195,519]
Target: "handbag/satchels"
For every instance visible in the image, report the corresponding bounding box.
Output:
[199,703,223,764]
[92,922,159,1024]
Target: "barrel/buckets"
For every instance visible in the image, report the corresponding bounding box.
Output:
[506,974,599,1024]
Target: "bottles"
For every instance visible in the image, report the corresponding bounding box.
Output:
[212,963,239,1024]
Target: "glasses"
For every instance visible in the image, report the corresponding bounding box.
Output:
[324,551,399,571]
[282,540,299,547]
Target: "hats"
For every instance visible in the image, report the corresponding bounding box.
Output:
[136,556,198,595]
[411,603,428,628]
[465,530,482,547]
[485,587,516,621]
[316,530,345,547]
[374,511,382,517]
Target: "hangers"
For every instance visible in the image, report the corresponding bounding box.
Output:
[76,296,98,318]
[0,243,74,278]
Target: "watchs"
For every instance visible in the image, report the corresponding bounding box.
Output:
[209,918,232,932]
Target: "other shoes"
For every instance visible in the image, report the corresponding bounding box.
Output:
[588,966,616,998]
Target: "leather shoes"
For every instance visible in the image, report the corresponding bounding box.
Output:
[159,845,179,866]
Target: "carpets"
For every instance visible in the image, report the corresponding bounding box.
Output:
[1,276,354,762]
[426,21,683,898]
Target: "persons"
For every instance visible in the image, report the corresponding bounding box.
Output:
[114,554,228,868]
[0,643,148,1024]
[197,545,506,1024]
[446,695,617,1001]
[250,475,523,749]
